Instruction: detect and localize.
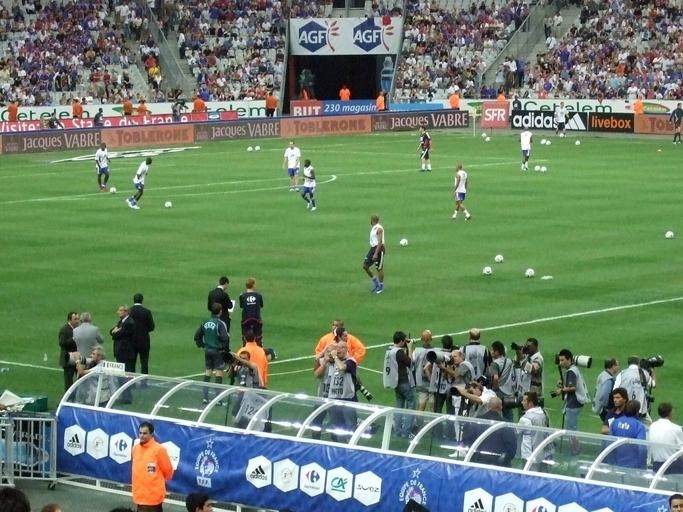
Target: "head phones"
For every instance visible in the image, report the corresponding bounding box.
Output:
[333,327,341,342]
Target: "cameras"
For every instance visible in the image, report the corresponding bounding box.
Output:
[548,390,560,399]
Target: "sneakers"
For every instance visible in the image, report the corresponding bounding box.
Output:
[307,201,312,209]
[214,401,225,407]
[520,165,529,170]
[289,187,300,192]
[310,206,317,211]
[99,187,109,193]
[464,214,471,222]
[372,285,385,295]
[125,199,140,210]
[418,169,433,173]
[201,397,212,404]
[672,140,682,145]
[395,426,457,445]
[448,215,456,221]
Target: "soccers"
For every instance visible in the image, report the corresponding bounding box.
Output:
[495,254,504,264]
[664,231,673,238]
[164,201,172,209]
[255,146,260,151]
[110,187,116,194]
[525,268,535,278]
[575,140,580,145]
[540,139,551,145]
[482,132,490,141]
[483,266,492,276]
[560,132,566,138]
[534,165,547,172]
[247,147,253,152]
[399,239,408,247]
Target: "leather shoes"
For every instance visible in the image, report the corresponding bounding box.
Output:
[117,398,133,404]
[128,382,153,389]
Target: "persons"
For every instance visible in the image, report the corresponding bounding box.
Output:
[94,142,111,193]
[1,0,683,134]
[42,503,63,512]
[383,328,683,477]
[300,159,319,213]
[280,140,301,193]
[311,319,366,444]
[124,156,151,210]
[0,488,31,511]
[184,493,213,512]
[129,422,174,511]
[57,293,155,407]
[669,493,682,512]
[518,122,532,169]
[361,214,386,292]
[667,103,682,145]
[415,124,434,172]
[451,163,469,221]
[194,276,278,432]
